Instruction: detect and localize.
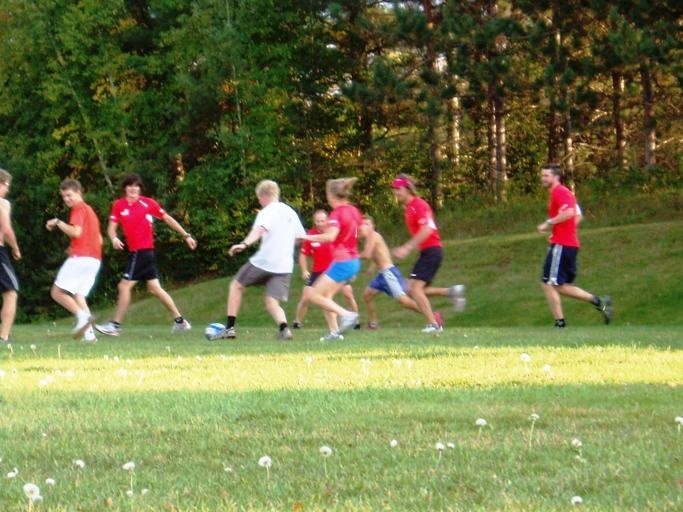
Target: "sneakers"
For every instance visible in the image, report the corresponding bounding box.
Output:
[320,332,344,344]
[219,327,236,339]
[452,284,467,313]
[169,319,192,333]
[434,311,446,328]
[337,310,359,335]
[83,324,98,344]
[69,314,92,338]
[91,322,122,337]
[419,324,444,334]
[274,327,294,341]
[596,294,612,325]
[365,325,380,330]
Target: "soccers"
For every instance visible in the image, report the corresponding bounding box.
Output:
[205,322,226,342]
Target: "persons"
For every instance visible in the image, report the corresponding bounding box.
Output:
[356,216,444,332]
[45,180,102,343]
[537,166,613,329]
[293,208,362,333]
[93,175,197,338]
[0,169,21,342]
[392,175,465,334]
[222,180,307,340]
[303,175,365,341]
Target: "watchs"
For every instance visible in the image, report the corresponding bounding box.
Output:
[239,241,249,247]
[546,218,553,228]
[182,233,193,240]
[53,218,59,226]
